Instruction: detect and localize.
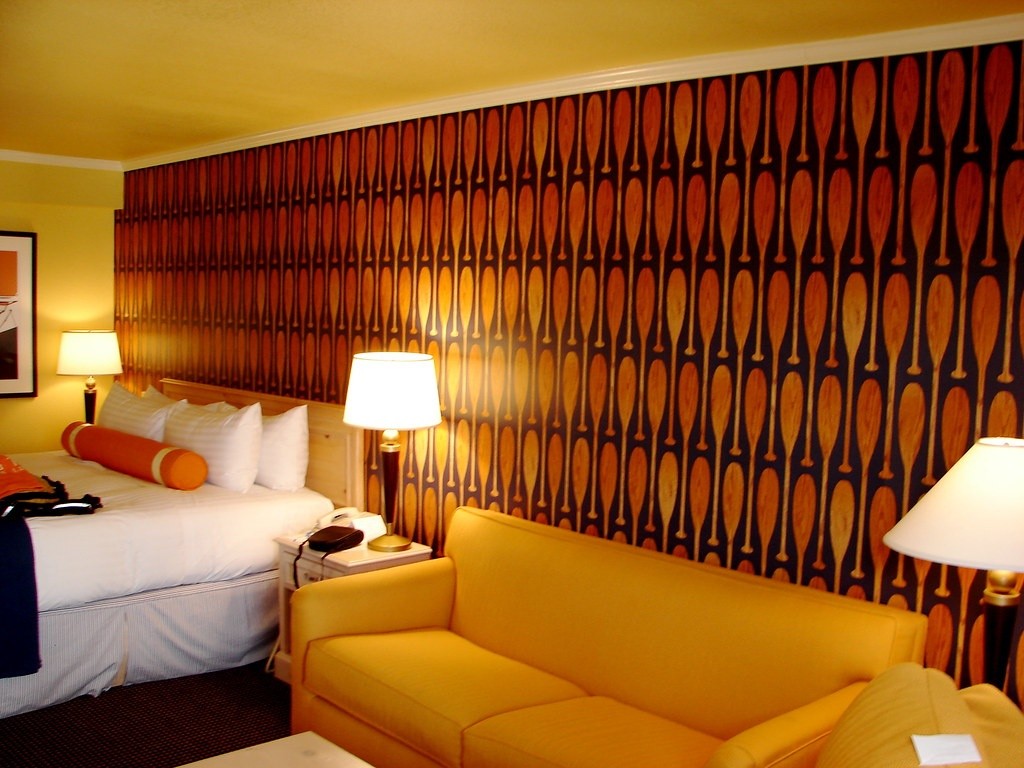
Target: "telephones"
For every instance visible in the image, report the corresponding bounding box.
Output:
[315,506,387,547]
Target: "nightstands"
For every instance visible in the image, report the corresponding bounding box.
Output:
[272,514,433,686]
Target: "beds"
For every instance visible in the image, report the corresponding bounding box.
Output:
[0,378,364,719]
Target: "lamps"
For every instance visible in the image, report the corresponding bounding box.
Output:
[56,329,123,424]
[882,436,1024,692]
[342,351,442,553]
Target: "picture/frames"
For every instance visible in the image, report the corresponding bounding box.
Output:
[0,230,38,399]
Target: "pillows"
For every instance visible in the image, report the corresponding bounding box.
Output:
[163,401,263,492]
[142,384,224,413]
[61,421,208,491]
[215,401,309,492]
[95,380,186,442]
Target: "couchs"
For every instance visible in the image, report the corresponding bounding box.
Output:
[814,661,1024,768]
[295,506,927,768]
